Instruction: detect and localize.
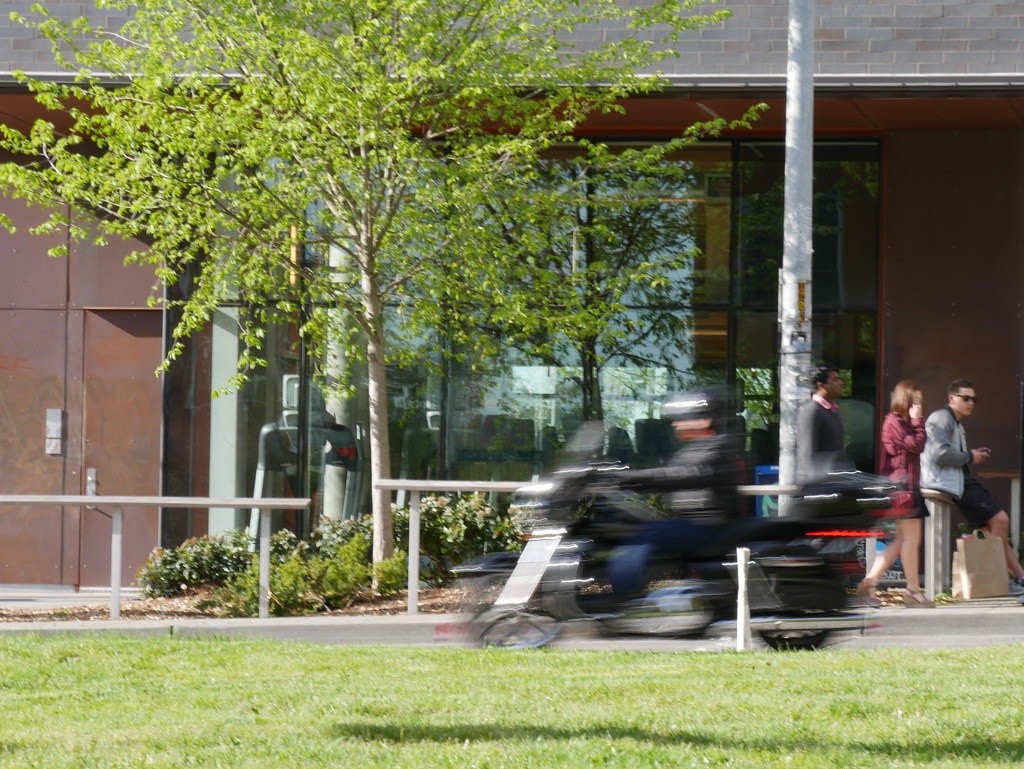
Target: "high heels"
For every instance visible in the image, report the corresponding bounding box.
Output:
[903,588,935,608]
[856,580,881,607]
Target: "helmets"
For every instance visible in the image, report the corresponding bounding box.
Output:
[659,387,730,420]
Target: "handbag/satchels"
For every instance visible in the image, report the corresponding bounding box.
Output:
[868,491,912,517]
[952,528,1010,599]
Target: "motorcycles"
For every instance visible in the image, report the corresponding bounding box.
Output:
[431,421,905,663]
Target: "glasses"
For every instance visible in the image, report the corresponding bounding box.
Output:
[954,393,977,402]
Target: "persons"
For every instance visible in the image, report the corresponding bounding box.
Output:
[580,388,748,614]
[856,379,936,610]
[919,378,1024,587]
[795,366,850,482]
